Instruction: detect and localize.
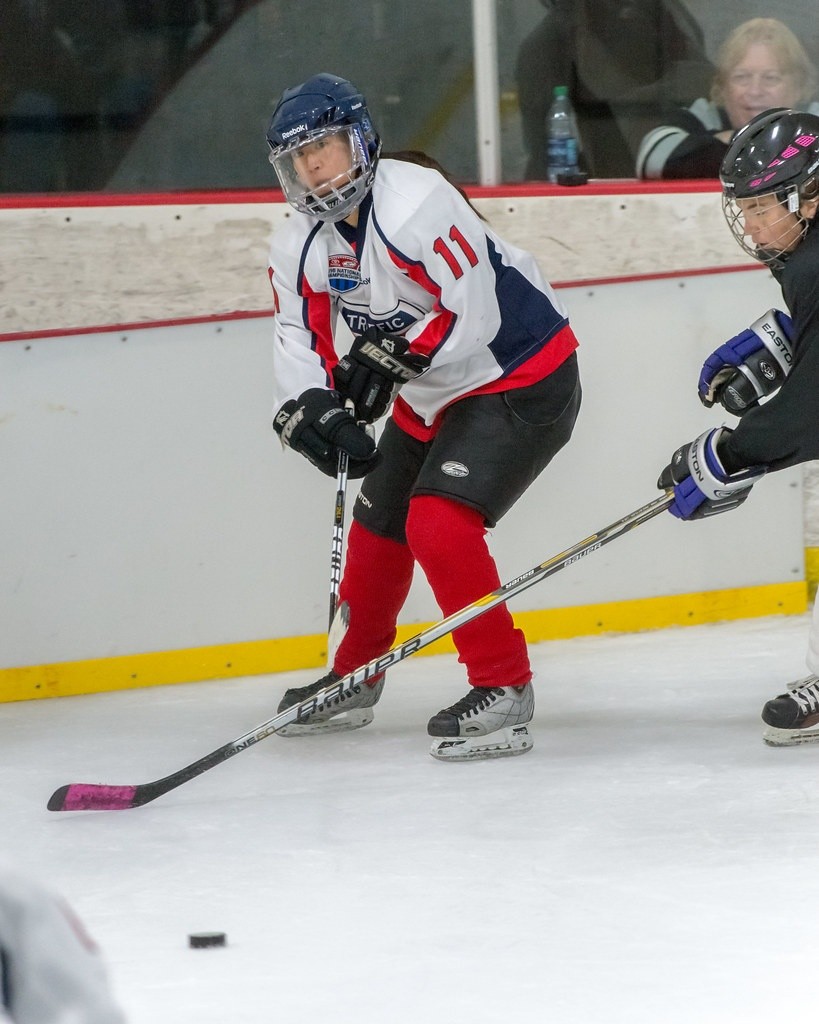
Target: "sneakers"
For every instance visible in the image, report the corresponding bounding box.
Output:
[274,668,384,736]
[428,684,535,761]
[759,674,818,746]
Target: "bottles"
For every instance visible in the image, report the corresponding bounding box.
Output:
[544,84,581,184]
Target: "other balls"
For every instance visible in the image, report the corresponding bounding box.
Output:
[188,934,228,947]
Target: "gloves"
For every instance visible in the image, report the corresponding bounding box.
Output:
[278,386,385,478]
[329,328,429,424]
[698,308,791,416]
[660,427,767,520]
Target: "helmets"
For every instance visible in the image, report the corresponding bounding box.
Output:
[265,70,380,222]
[720,106,819,268]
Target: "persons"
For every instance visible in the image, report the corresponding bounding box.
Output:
[517,0,707,184]
[634,16,818,178]
[261,74,579,742]
[656,109,818,727]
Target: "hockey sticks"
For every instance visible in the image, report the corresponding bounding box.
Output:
[273,400,376,738]
[44,484,680,811]
